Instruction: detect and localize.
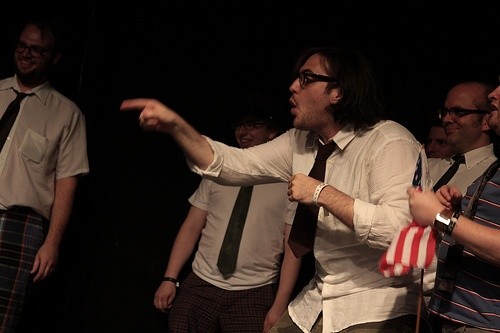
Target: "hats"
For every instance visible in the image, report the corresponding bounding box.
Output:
[233,87,291,128]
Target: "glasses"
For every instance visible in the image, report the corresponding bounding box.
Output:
[296,70,343,88]
[438,106,491,122]
[235,120,271,129]
[16,43,51,58]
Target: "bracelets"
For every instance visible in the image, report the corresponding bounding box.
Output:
[447,213,461,234]
[313,182,330,206]
[161,276,180,287]
[435,207,454,230]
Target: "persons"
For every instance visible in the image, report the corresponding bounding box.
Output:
[0,20,89,333]
[155,81,499,333]
[120,47,438,333]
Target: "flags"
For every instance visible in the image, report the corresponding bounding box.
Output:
[380,146,438,278]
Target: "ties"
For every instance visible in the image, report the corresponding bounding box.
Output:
[217,185,253,280]
[433,155,465,192]
[287,139,337,258]
[0,87,35,152]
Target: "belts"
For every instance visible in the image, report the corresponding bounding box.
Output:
[8,204,31,214]
